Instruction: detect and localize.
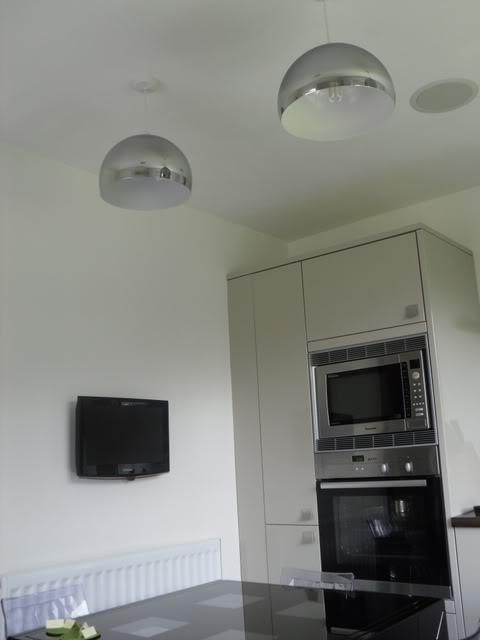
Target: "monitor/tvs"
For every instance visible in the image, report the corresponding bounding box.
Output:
[74,394,169,480]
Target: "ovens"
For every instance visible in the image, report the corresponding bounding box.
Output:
[305,330,440,450]
[309,444,455,602]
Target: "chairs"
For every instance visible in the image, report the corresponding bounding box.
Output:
[0,582,91,638]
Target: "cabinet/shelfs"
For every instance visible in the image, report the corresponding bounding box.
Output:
[227,222,478,640]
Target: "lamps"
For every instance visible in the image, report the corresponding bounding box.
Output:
[276,0,399,143]
[98,80,194,211]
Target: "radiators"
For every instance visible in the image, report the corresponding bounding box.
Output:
[0,536,223,640]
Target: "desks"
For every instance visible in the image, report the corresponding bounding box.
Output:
[7,579,447,640]
